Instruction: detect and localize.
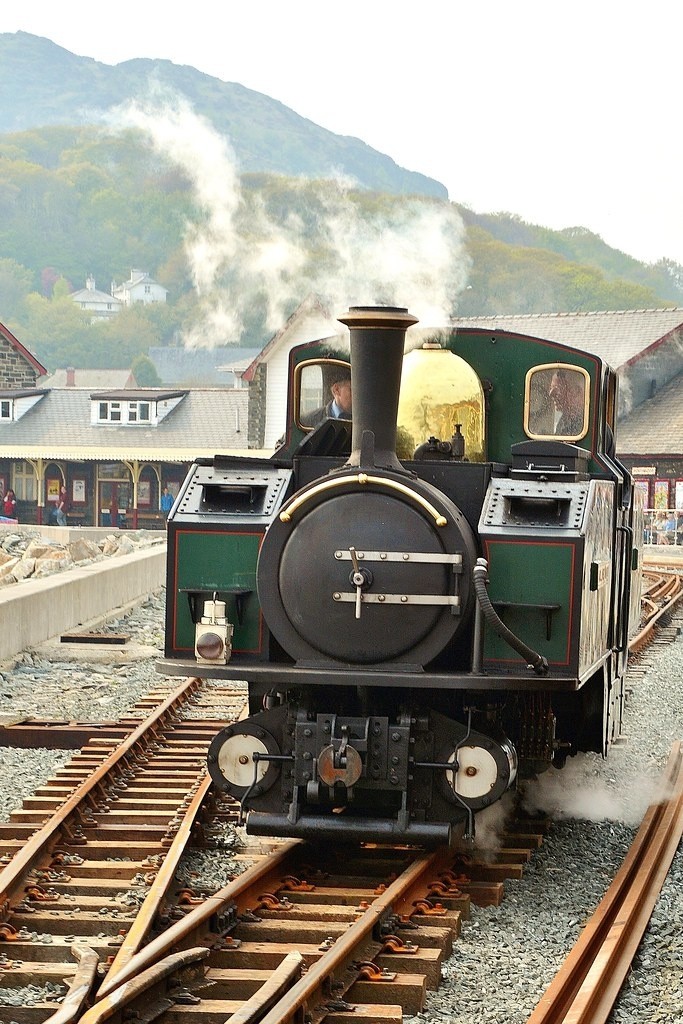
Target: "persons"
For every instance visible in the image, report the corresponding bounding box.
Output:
[49,486,68,525]
[3,489,20,519]
[549,369,615,457]
[161,487,175,530]
[643,511,683,545]
[275,370,352,451]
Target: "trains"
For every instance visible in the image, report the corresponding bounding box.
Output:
[155,306,644,846]
